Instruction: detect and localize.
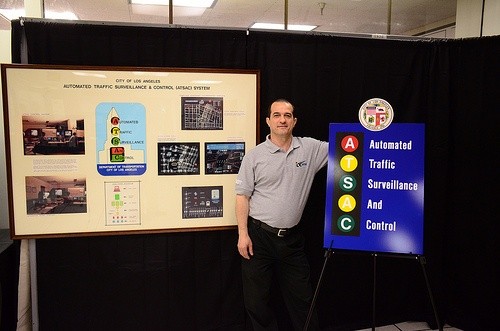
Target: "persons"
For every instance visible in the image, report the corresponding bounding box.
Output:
[233,97,329,331]
[40,132,48,154]
[68,132,78,151]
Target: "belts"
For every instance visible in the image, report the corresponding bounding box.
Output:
[249,217,300,237]
